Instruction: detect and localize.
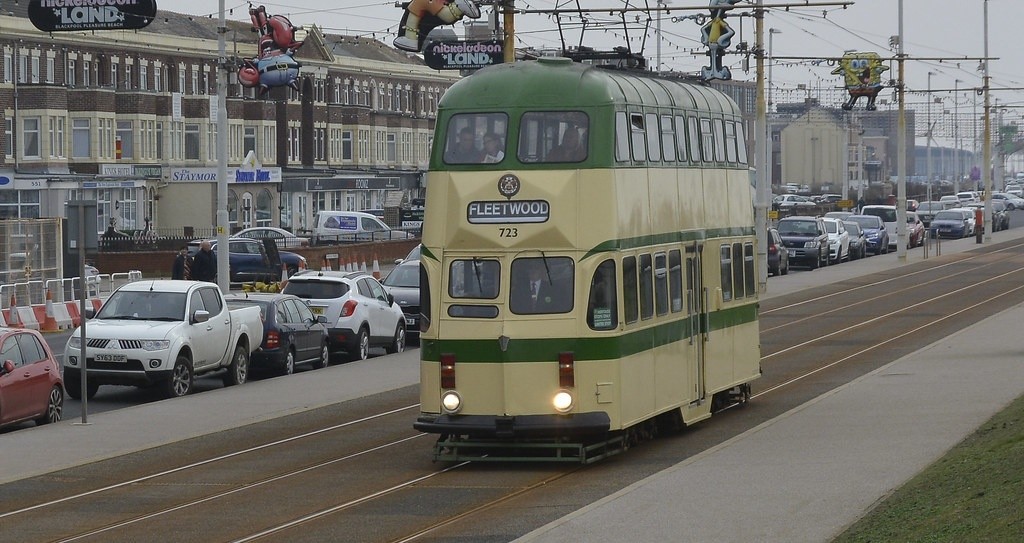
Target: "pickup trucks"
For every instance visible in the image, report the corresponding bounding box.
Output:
[63,279,265,401]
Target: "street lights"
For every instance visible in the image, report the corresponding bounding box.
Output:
[954,79,964,196]
[927,71,938,199]
[657,0,674,73]
[973,88,1006,189]
[769,27,783,226]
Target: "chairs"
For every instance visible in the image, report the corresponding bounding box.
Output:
[784,225,817,232]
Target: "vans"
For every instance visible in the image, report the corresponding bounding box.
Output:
[314,210,413,244]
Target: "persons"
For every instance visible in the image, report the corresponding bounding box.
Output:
[193,241,217,283]
[172,244,197,280]
[478,133,505,162]
[444,127,481,163]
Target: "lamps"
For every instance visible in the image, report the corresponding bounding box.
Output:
[241,205,251,212]
[116,200,119,210]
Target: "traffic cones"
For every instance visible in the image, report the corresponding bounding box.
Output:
[43,290,58,330]
[7,296,20,327]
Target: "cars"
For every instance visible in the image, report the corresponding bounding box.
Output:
[823,199,927,264]
[0,327,65,430]
[916,170,1024,238]
[7,253,102,284]
[766,228,789,275]
[224,290,332,378]
[231,227,310,252]
[773,189,844,214]
[380,259,423,335]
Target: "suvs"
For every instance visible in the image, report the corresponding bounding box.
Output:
[182,238,306,286]
[776,214,830,268]
[282,269,408,362]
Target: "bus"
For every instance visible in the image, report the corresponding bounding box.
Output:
[413,55,761,464]
